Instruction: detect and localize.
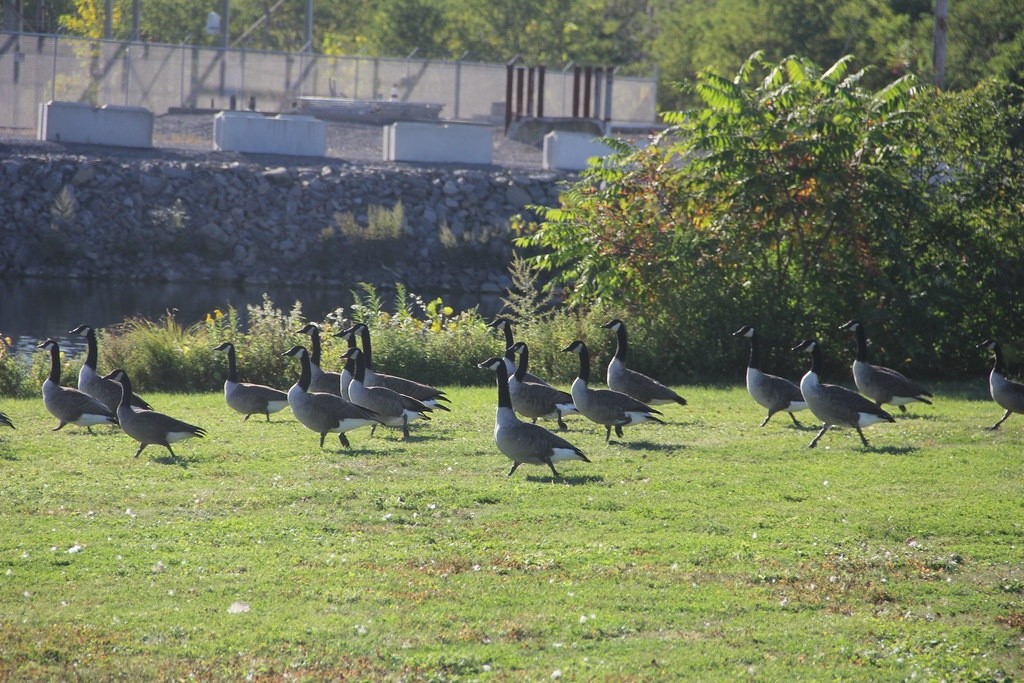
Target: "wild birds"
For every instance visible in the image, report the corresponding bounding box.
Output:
[0,308,1024,488]
